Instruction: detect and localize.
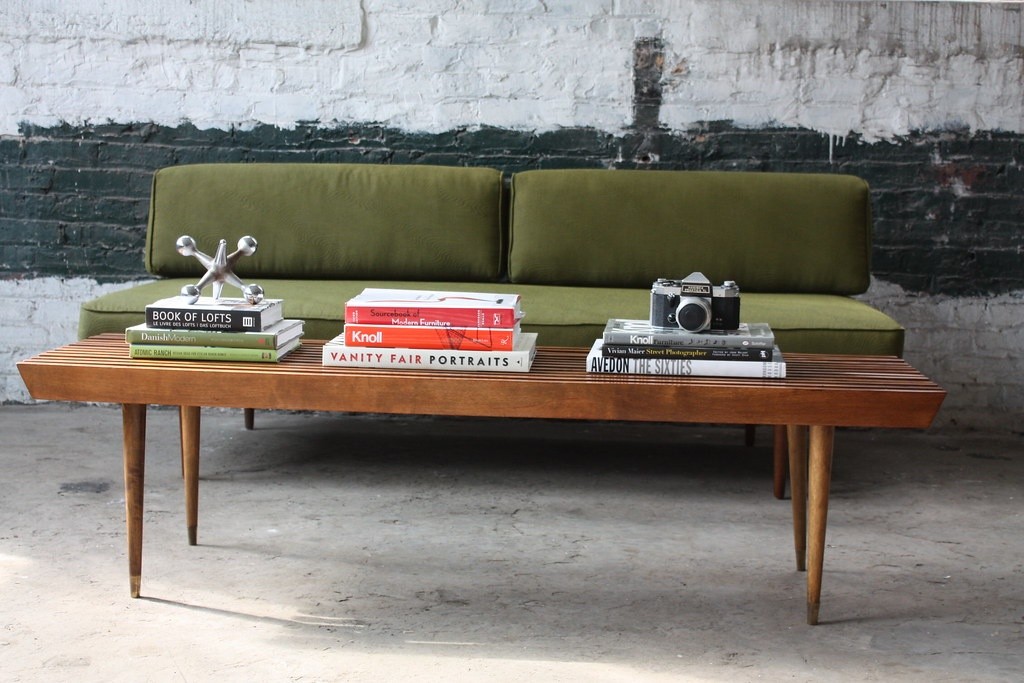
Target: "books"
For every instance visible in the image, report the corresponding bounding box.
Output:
[126,296,306,363]
[322,288,538,372]
[586,318,787,379]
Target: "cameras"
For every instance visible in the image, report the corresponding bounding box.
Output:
[650,271,741,334]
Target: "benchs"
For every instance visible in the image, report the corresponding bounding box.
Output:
[77,162,908,501]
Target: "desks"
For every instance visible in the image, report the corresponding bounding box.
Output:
[17,331,948,626]
